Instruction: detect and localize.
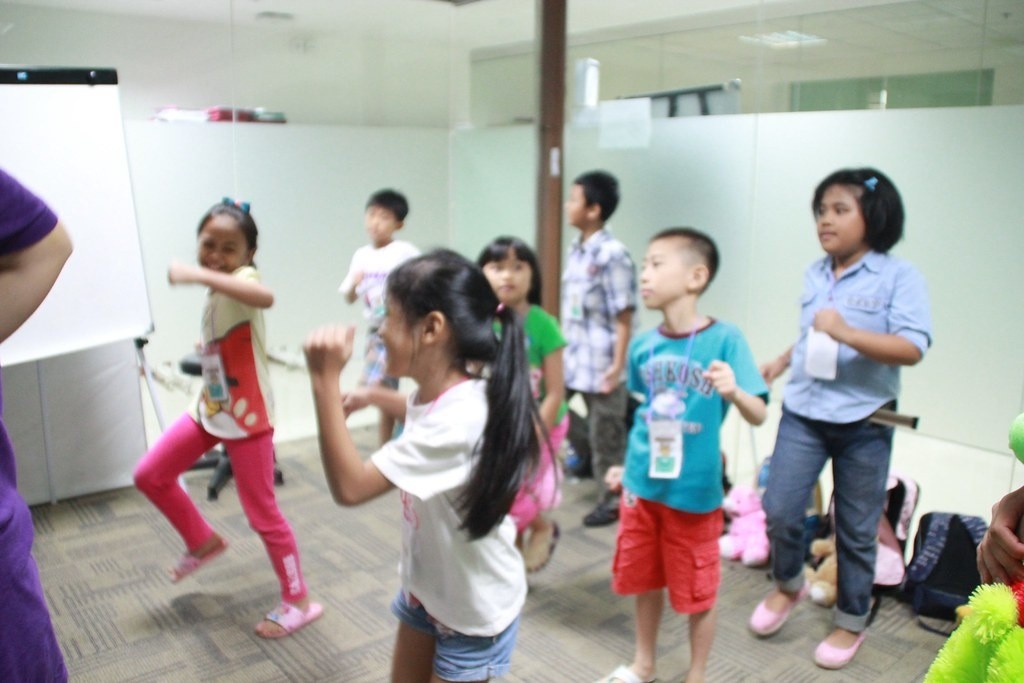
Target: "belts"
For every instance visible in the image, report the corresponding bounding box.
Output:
[870,404,919,430]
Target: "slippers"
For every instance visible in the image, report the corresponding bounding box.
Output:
[255,596,321,637]
[598,664,655,682]
[168,540,228,585]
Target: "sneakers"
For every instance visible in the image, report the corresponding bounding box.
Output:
[583,484,622,527]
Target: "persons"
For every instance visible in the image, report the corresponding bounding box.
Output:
[339,189,422,450]
[603,228,770,683]
[561,170,637,528]
[748,168,932,670]
[0,167,72,683]
[134,195,322,640]
[474,237,570,574]
[305,249,559,683]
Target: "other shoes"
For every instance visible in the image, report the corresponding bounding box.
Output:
[525,521,559,574]
[750,578,810,635]
[816,625,868,668]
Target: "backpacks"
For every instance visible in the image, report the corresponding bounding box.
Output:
[904,512,988,621]
[828,470,919,626]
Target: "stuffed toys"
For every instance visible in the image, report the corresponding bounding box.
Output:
[811,531,837,607]
[718,481,769,566]
